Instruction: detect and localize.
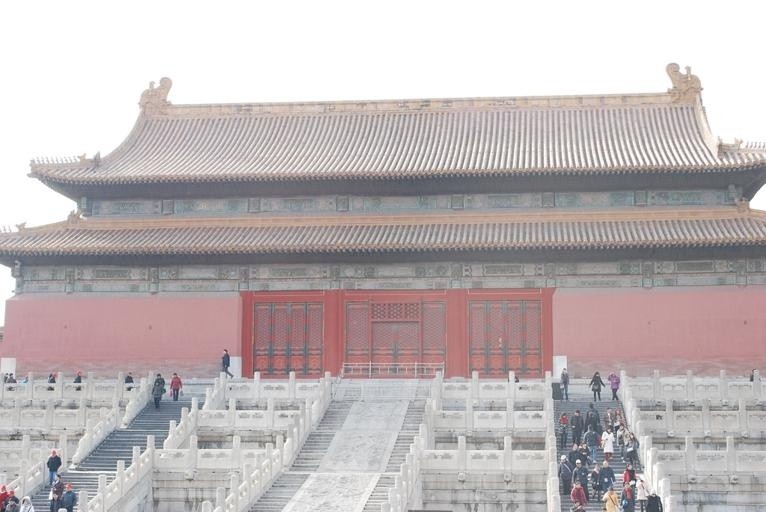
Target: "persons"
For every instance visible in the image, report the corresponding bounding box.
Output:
[125,372,133,391]
[4,372,28,390]
[508,376,519,382]
[47,370,56,390]
[555,368,662,512]
[73,372,81,391]
[0,450,77,512]
[750,369,761,381]
[151,382,162,409]
[222,349,234,379]
[155,374,165,394]
[170,373,182,401]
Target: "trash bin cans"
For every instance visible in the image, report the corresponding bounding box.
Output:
[552,383,564,400]
